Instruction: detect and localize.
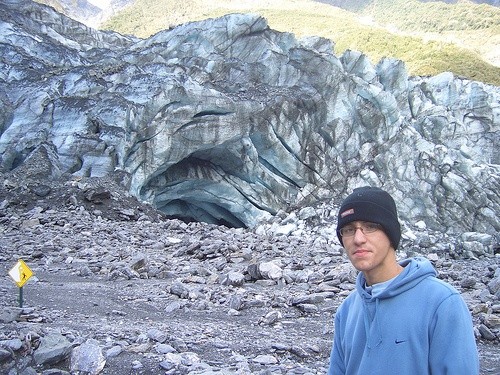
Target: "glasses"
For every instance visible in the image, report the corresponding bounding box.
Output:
[336,223,381,237]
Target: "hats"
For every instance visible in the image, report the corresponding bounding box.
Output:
[336,186,400,250]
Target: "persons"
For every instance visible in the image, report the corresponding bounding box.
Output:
[327,185,481,375]
